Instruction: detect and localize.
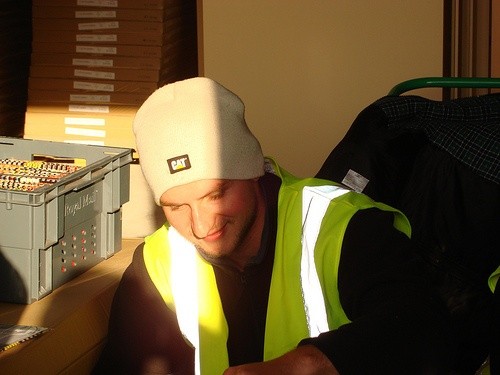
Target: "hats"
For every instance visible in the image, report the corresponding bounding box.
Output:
[134,77,265,208]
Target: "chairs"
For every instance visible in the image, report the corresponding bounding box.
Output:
[313,77,499,270]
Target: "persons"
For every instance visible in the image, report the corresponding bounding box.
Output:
[96,77,432,375]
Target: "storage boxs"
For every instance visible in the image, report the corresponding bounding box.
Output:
[0,135,137,250]
[0,206,122,304]
